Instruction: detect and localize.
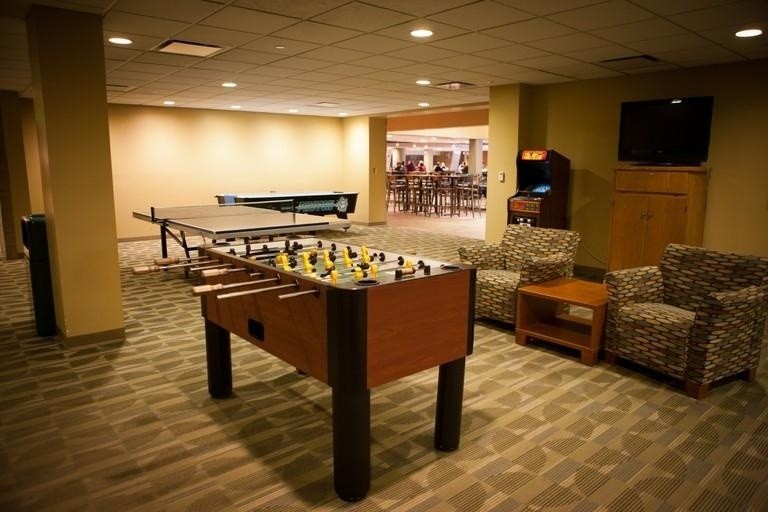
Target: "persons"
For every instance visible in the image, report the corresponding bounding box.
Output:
[482,160,487,177]
[392,160,468,188]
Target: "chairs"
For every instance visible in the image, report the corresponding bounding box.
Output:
[387,170,481,219]
[457,221,582,331]
[602,241,767,401]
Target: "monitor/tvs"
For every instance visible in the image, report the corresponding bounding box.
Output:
[530,182,551,192]
[617,95,714,168]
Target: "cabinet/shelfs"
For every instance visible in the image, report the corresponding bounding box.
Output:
[607,164,711,273]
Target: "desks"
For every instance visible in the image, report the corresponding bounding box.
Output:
[132,191,360,282]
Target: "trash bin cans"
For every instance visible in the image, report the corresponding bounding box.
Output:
[29,213,58,337]
